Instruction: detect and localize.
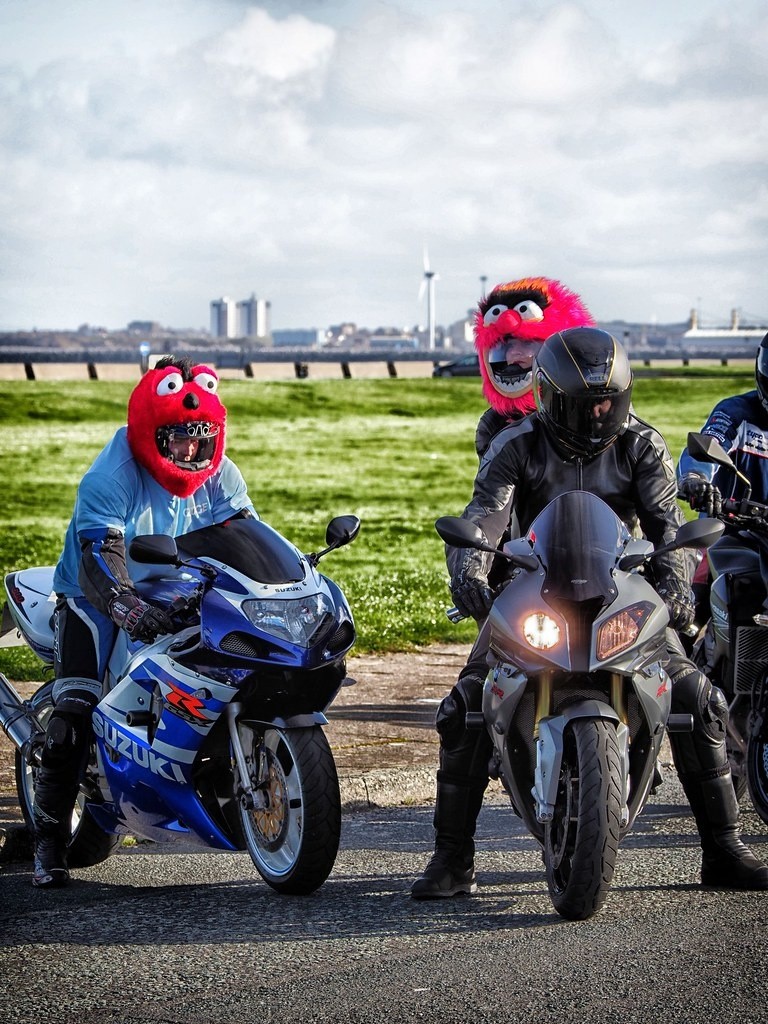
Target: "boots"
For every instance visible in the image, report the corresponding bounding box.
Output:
[409,773,481,901]
[30,764,81,885]
[676,762,768,888]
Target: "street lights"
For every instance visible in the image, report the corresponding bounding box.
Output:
[424,270,435,352]
[478,275,489,300]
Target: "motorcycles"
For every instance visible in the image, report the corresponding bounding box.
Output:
[677,427,768,828]
[0,513,364,900]
[429,487,726,924]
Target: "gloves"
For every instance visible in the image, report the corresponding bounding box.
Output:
[657,580,703,629]
[110,595,172,646]
[682,479,723,517]
[444,565,496,617]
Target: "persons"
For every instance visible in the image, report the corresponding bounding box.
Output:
[676,331,768,624]
[475,341,540,459]
[32,428,260,886]
[411,322,768,903]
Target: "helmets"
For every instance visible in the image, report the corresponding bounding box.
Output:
[533,324,635,458]
[472,277,599,420]
[127,356,228,500]
[754,332,768,411]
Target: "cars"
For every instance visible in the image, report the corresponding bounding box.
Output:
[431,354,482,379]
